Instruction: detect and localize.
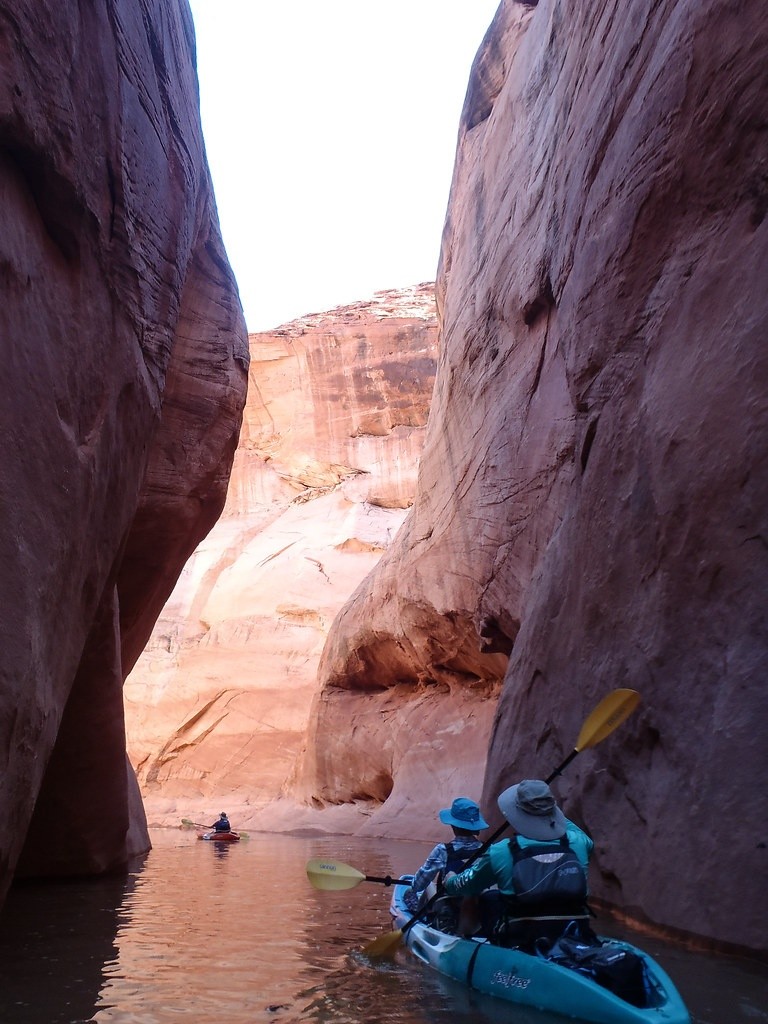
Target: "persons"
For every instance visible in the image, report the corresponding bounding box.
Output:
[443,780,593,926]
[207,812,231,832]
[403,797,490,917]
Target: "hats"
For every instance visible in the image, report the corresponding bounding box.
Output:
[439,797,489,830]
[498,779,567,840]
[219,812,228,820]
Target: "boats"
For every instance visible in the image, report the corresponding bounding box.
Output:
[196,830,241,840]
[387,869,694,1024]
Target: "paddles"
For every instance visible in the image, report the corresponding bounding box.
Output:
[359,685,645,958]
[305,858,419,893]
[181,819,250,838]
